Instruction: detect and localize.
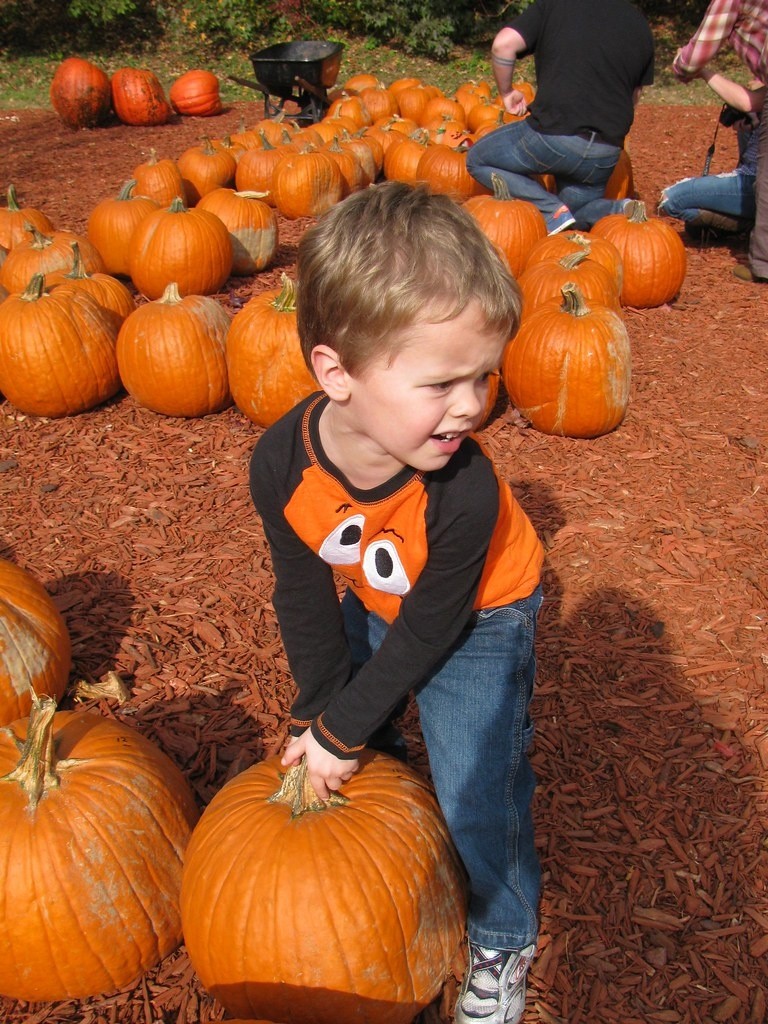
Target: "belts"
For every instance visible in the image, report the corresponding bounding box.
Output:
[579,127,620,149]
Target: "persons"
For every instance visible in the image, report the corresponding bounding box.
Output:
[462,0,656,239]
[671,0,768,283]
[656,66,767,243]
[244,179,548,1024]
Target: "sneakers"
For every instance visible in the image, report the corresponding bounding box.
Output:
[453,937,537,1022]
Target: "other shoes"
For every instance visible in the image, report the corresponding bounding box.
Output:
[733,265,756,282]
[543,203,575,241]
[620,198,637,220]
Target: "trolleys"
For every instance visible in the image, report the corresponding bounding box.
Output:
[227,41,345,130]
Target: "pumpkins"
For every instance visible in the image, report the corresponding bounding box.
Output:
[0,691,199,1002]
[0,57,686,438]
[186,753,465,1024]
[0,556,71,729]
